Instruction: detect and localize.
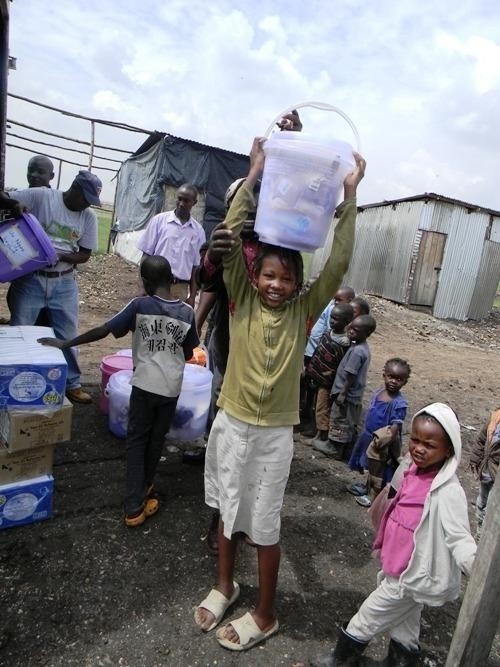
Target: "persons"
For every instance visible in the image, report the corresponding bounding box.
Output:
[196,108,303,558]
[1,167,93,405]
[190,134,368,650]
[36,253,204,529]
[294,283,377,460]
[4,151,53,323]
[307,401,480,667]
[346,355,413,507]
[469,404,500,544]
[136,180,204,308]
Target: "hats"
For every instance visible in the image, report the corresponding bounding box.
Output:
[222,176,263,207]
[73,168,104,208]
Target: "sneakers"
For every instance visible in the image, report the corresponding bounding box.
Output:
[124,497,159,526]
[355,494,374,506]
[145,482,156,496]
[346,481,367,494]
[182,447,205,461]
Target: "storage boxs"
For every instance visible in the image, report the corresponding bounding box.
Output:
[1,324,68,407]
[1,475,54,530]
[0,396,73,454]
[0,441,56,486]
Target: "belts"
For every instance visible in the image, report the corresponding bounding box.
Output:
[170,277,192,284]
[32,264,77,279]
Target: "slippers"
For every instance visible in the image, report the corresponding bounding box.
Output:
[215,611,281,651]
[193,574,243,632]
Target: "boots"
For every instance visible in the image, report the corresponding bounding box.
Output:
[292,399,355,462]
[375,637,423,667]
[310,620,372,667]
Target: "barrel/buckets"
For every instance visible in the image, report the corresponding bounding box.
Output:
[164,363,214,441]
[104,369,134,440]
[98,355,133,416]
[0,210,60,284]
[254,100,363,254]
[185,347,208,367]
[116,348,132,356]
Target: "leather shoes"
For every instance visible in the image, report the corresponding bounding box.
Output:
[245,533,257,546]
[69,388,93,404]
[207,510,222,550]
